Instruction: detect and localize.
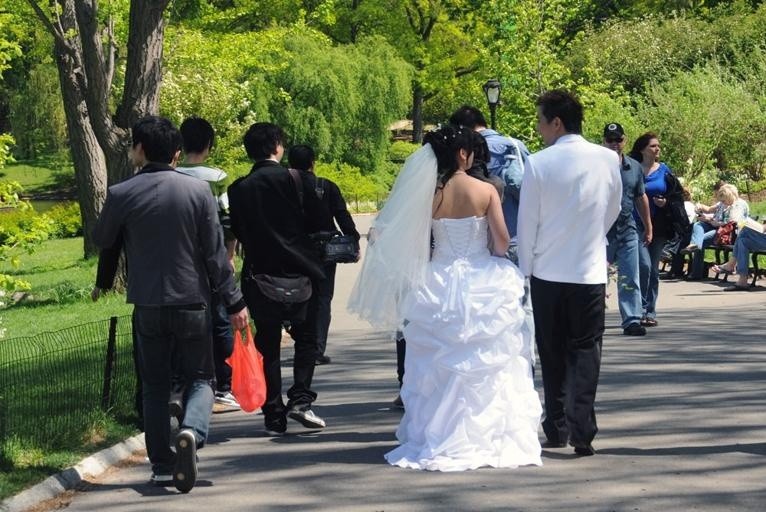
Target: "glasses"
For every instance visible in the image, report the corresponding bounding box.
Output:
[126,140,133,149]
[606,138,625,143]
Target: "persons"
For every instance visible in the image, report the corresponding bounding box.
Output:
[348,91,624,471]
[659,180,766,291]
[599,122,684,336]
[92,116,361,492]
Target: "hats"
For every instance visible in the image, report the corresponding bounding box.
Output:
[604,123,624,138]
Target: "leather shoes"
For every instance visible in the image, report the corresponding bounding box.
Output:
[315,354,329,365]
[681,245,698,254]
[168,392,185,427]
[686,273,702,280]
[569,440,596,457]
[545,439,566,448]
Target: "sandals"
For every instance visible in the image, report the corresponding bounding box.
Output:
[647,317,657,326]
[641,317,647,325]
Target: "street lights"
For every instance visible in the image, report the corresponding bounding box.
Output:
[482,76,503,132]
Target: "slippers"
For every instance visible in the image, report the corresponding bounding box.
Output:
[712,264,733,275]
[724,284,750,291]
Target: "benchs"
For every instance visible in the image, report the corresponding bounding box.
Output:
[661,216,766,286]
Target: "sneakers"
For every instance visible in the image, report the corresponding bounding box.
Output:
[392,395,404,408]
[661,250,673,262]
[214,390,240,406]
[288,403,326,428]
[263,423,284,437]
[151,462,174,480]
[660,271,684,279]
[173,428,198,493]
[624,323,646,335]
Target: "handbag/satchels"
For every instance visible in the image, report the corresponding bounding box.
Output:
[498,136,525,201]
[243,273,313,323]
[713,221,738,245]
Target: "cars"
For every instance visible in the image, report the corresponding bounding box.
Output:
[386,118,439,146]
